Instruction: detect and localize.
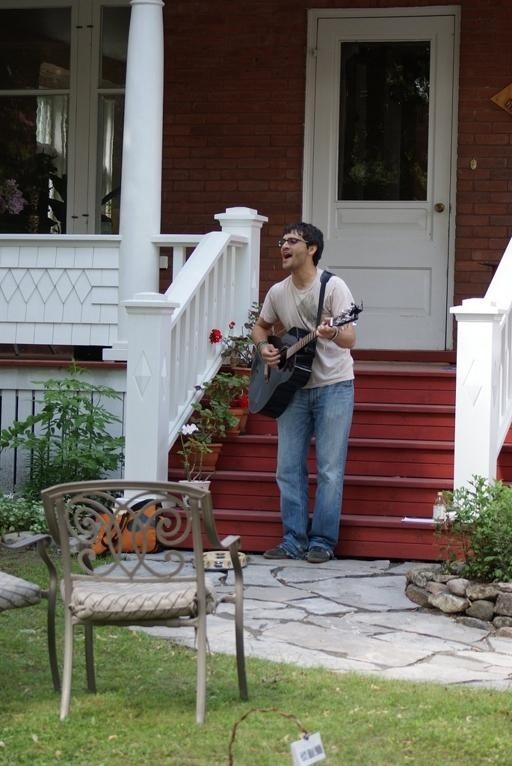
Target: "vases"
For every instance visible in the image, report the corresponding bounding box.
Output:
[179,481,211,509]
[233,367,252,378]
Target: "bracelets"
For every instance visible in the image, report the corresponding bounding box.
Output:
[329,326,339,343]
[256,340,269,360]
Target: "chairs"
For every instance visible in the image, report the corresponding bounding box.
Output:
[1,531,61,695]
[42,480,248,723]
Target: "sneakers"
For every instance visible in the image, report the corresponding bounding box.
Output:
[263,544,296,559]
[306,543,332,562]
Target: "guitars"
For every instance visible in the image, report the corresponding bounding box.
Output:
[248,301,363,421]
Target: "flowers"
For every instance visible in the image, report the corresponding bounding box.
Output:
[177,424,213,481]
[209,322,254,368]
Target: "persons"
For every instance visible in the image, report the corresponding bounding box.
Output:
[251,222,362,564]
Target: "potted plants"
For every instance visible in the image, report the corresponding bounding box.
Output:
[187,400,240,471]
[196,373,244,434]
[240,375,251,433]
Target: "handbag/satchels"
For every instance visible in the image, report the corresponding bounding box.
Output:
[84,497,164,560]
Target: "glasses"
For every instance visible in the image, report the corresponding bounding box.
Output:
[277,237,311,247]
